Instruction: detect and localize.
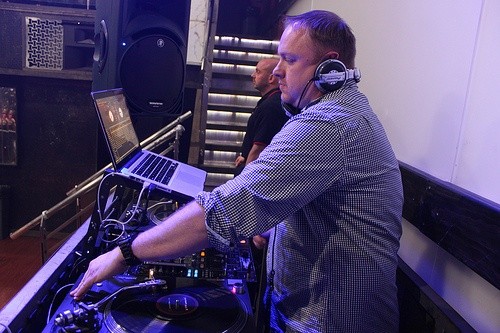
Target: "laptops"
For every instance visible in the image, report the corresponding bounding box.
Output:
[90,87,207,203]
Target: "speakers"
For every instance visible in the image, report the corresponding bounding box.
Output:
[92,0,192,116]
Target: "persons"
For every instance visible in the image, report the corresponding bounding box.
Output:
[234,56,291,251]
[0,108,15,131]
[69,10,403,333]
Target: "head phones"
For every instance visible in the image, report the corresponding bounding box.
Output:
[313,58,361,95]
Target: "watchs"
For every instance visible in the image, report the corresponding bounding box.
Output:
[116,232,142,268]
